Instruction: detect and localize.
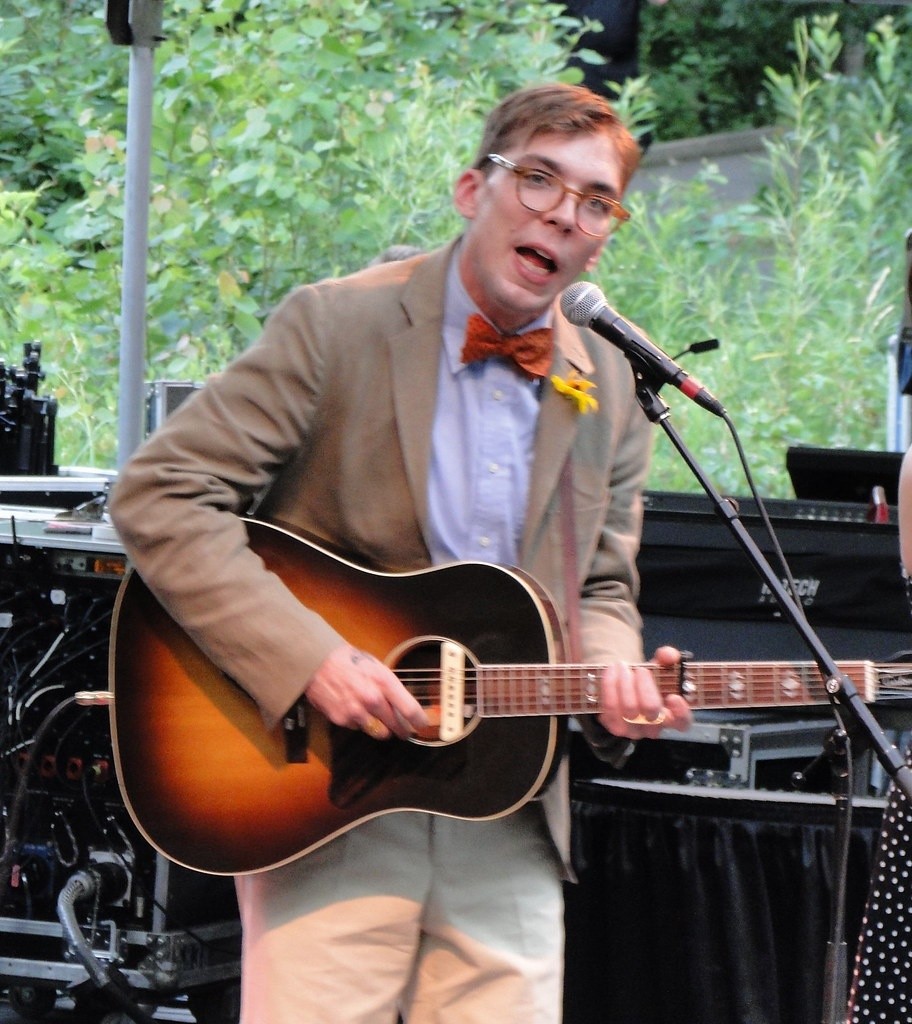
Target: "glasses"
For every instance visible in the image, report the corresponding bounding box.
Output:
[485,152,632,242]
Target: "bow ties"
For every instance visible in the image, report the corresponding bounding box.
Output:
[457,312,556,378]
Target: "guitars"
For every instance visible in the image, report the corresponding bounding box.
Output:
[107,512,912,878]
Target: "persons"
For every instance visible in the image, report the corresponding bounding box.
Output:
[109,81,697,1019]
[843,281,912,1024]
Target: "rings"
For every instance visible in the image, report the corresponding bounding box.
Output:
[624,709,667,726]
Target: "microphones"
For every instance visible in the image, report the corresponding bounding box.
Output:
[555,281,730,425]
